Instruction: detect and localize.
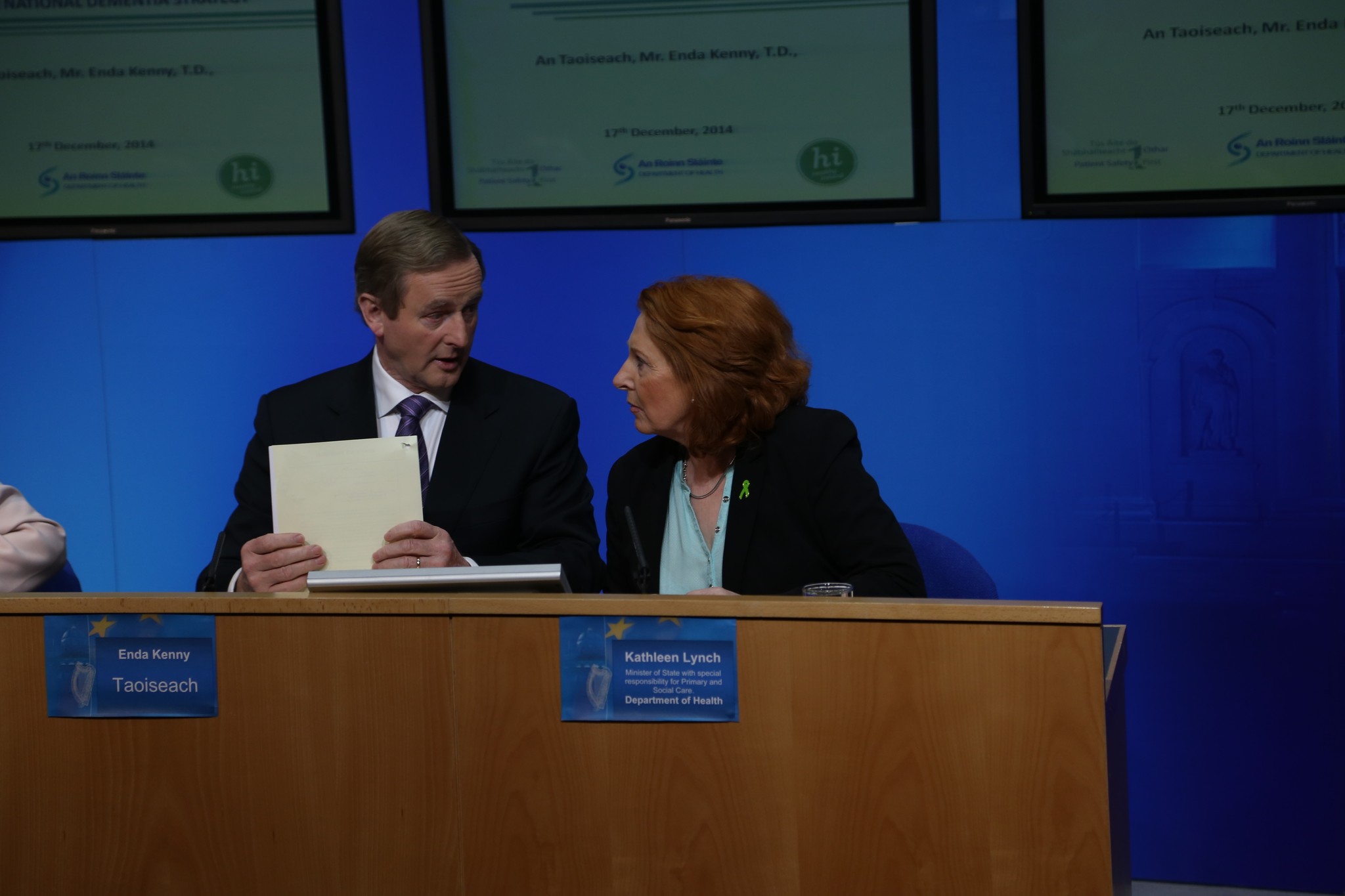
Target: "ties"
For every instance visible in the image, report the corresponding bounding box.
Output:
[395,395,429,492]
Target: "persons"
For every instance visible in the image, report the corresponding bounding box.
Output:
[0,483,68,593]
[196,211,608,594]
[607,276,927,597]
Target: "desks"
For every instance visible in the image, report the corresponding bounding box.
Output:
[0,593,1113,896]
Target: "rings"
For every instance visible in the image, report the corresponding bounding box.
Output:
[416,555,420,569]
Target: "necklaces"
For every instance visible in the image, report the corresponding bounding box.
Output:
[682,458,726,498]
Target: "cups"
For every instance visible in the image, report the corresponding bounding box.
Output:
[802,583,853,598]
